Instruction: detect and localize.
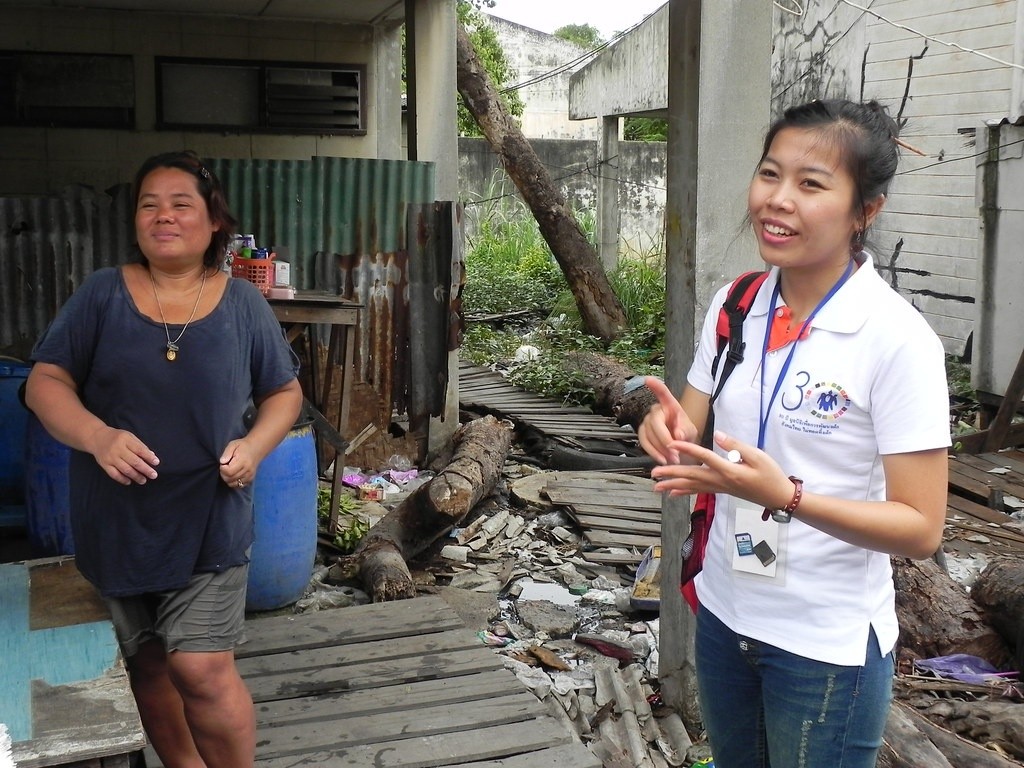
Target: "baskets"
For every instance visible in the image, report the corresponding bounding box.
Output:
[230,250,276,297]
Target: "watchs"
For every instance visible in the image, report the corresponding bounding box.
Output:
[762,476,804,524]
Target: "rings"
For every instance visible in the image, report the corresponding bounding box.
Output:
[237,479,245,489]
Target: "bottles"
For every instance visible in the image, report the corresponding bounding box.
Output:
[310,563,336,583]
[236,234,255,258]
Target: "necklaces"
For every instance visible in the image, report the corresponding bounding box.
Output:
[152,265,208,361]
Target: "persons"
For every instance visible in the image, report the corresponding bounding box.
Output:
[24,149,302,768]
[638,93,953,768]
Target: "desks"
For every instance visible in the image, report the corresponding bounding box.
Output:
[0,555,148,768]
[266,287,367,532]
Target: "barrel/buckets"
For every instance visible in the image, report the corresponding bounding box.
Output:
[242,415,317,610]
[0,360,77,563]
